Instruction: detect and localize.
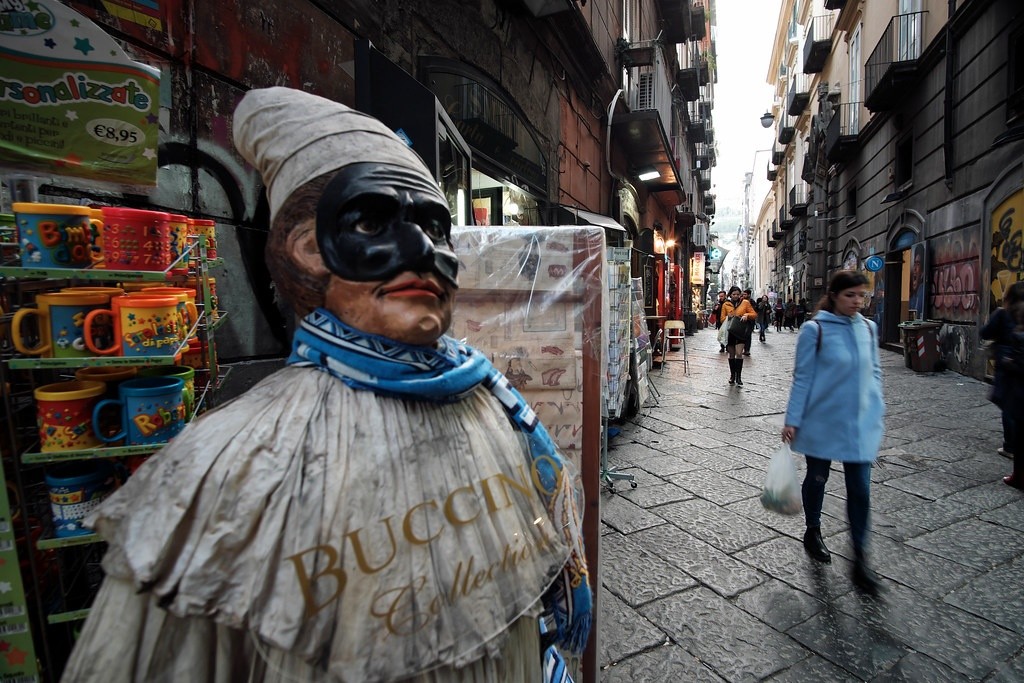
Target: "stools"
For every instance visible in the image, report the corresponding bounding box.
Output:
[661,320,691,376]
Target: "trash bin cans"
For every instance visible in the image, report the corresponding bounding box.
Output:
[897,319,944,372]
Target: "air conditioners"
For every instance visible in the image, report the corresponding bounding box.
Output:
[638,72,654,110]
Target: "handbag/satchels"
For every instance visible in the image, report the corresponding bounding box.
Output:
[728,316,750,341]
[760,442,803,514]
[717,316,729,347]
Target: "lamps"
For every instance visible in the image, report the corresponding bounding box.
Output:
[639,165,660,181]
[761,109,775,128]
[880,191,908,205]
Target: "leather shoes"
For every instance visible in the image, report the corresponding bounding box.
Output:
[852,569,879,591]
[804,527,832,562]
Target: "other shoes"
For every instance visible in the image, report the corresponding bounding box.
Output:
[1003,474,1015,482]
[759,326,794,342]
[744,352,750,355]
[998,448,1014,458]
[720,348,724,352]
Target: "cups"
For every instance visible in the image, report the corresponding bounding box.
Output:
[11,199,218,537]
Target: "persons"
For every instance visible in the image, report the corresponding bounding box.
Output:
[712,289,805,355]
[981,280,1024,491]
[721,286,757,385]
[59,85,592,683]
[781,270,883,598]
[909,243,924,319]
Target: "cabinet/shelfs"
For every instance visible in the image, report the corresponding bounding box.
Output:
[0,242,232,625]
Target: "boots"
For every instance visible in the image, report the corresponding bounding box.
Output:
[728,358,735,383]
[735,359,743,385]
